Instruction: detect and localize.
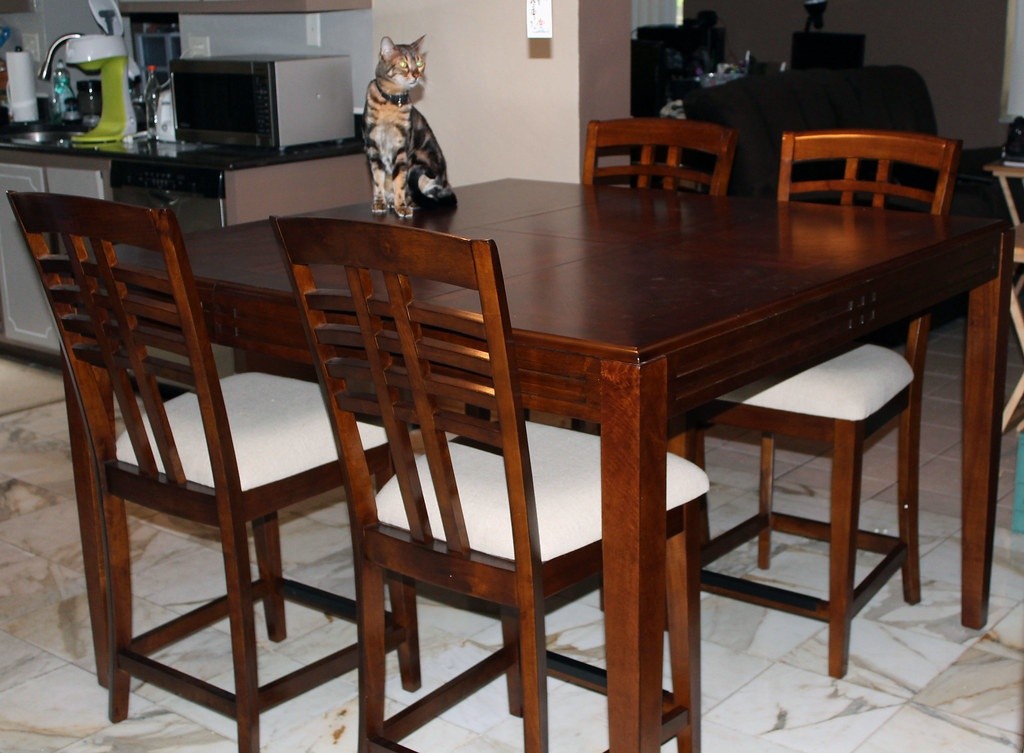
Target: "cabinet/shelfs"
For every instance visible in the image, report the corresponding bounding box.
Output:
[0,147,225,395]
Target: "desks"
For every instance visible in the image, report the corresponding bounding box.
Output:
[63,177,1016,753]
[983,158,1024,226]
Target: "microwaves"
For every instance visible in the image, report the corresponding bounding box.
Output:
[170,55,354,154]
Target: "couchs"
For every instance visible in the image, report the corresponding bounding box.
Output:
[686,65,1024,349]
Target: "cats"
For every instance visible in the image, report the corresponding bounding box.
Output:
[362,34,458,221]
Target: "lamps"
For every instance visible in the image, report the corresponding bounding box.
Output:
[802,0,827,32]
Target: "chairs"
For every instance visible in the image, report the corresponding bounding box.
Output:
[699,130,964,680]
[269,214,711,753]
[582,118,738,196]
[7,189,421,753]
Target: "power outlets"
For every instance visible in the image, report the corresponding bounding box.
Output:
[21,33,40,62]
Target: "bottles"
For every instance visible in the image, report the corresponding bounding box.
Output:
[52,59,75,125]
[143,65,162,141]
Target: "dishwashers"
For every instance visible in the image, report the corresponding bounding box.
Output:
[109,160,233,390]
